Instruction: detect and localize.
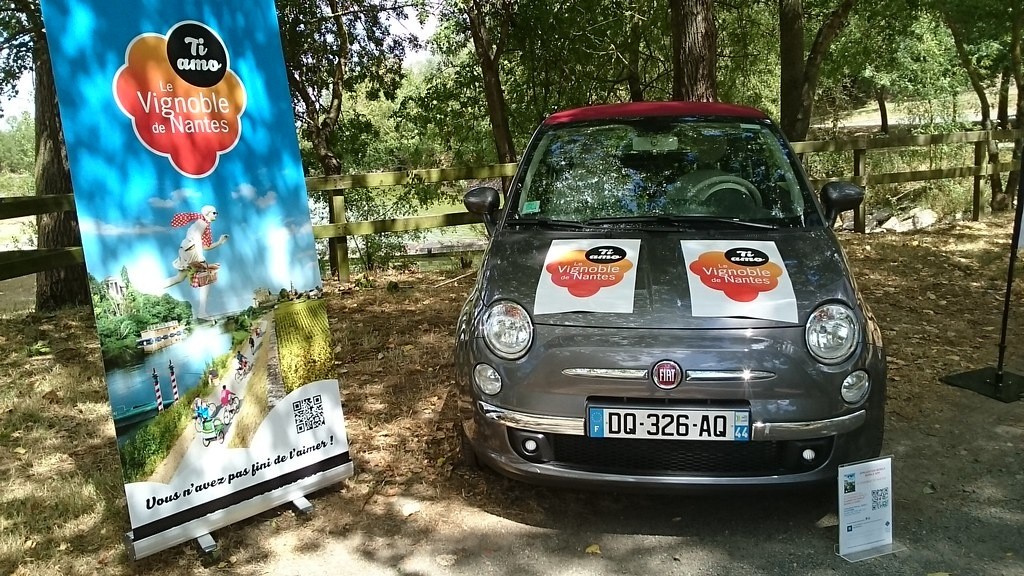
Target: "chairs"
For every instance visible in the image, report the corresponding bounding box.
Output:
[545,137,640,219]
[663,127,749,211]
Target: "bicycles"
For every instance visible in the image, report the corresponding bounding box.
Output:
[235,361,253,384]
[194,396,240,433]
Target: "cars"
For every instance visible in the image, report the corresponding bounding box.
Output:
[453,101,887,492]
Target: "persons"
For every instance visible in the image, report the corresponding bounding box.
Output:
[233,350,247,371]
[256,327,260,338]
[193,393,202,410]
[248,335,254,355]
[209,368,218,379]
[221,385,237,406]
[164,205,229,321]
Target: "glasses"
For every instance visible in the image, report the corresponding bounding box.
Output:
[210,212,219,215]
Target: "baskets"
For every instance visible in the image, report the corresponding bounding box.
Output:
[189,261,217,288]
